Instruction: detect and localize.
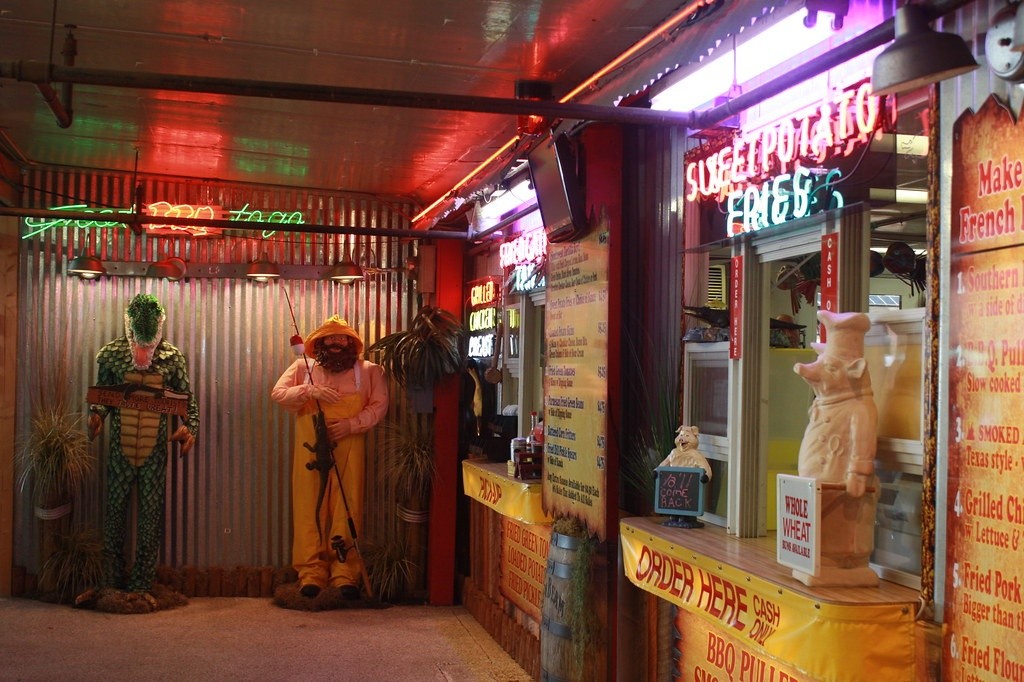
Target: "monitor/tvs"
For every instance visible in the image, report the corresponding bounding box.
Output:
[528,126,582,244]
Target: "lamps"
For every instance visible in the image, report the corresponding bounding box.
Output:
[246,238,281,282]
[145,253,186,280]
[329,240,364,284]
[489,183,508,197]
[66,233,105,280]
[870,0,980,98]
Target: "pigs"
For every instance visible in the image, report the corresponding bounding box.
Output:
[650,425,713,484]
[792,310,878,498]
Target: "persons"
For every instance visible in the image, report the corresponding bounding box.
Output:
[269,312,388,609]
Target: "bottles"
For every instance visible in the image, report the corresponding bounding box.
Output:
[511,411,544,464]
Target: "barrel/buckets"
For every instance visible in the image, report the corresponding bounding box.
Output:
[539,529,632,682]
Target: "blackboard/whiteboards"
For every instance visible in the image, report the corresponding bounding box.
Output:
[656,466,705,516]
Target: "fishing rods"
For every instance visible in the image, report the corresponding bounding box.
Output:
[280,284,376,602]
